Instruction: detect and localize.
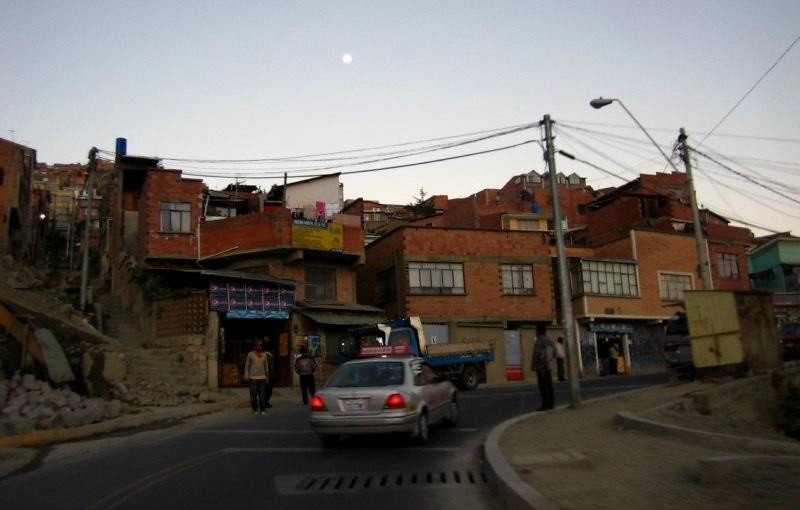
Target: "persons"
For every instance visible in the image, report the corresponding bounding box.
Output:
[555,336,567,383]
[294,345,317,406]
[608,342,620,376]
[309,337,319,357]
[243,336,273,417]
[530,322,558,412]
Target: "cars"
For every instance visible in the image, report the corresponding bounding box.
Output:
[308,356,459,445]
[778,322,800,361]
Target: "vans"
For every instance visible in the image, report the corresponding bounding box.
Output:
[663,311,692,365]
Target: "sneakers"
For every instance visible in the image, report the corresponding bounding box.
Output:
[254,404,272,416]
[536,406,554,411]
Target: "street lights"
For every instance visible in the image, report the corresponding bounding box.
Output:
[590,98,713,291]
